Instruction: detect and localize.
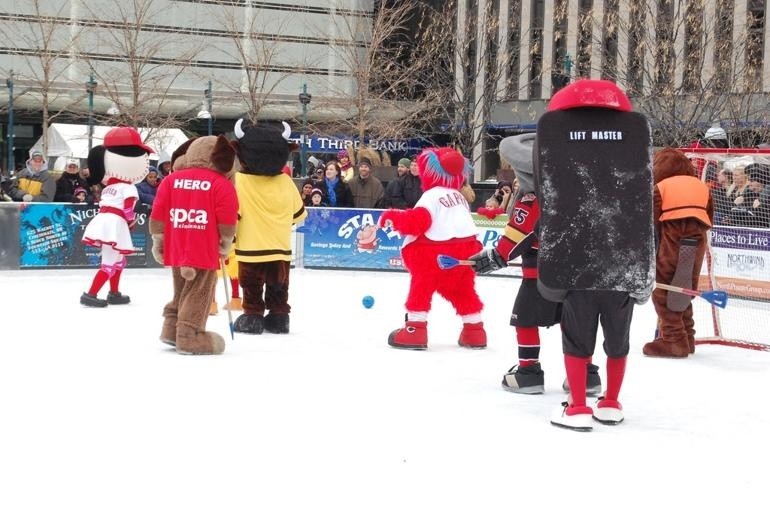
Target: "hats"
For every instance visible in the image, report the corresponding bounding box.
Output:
[301,149,416,199]
[66,158,79,175]
[74,187,87,197]
[29,151,43,163]
[149,167,158,174]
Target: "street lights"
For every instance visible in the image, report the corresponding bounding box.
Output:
[6,79,16,173]
[197,82,214,135]
[86,68,97,151]
[298,83,311,176]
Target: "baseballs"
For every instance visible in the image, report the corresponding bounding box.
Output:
[362,296,375,308]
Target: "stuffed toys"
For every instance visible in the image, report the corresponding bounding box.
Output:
[470,132,603,396]
[148,135,239,356]
[234,116,308,335]
[209,234,244,315]
[377,147,489,350]
[533,80,658,432]
[642,146,714,358]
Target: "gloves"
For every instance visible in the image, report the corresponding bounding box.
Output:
[22,194,32,202]
[86,194,94,206]
[70,195,78,203]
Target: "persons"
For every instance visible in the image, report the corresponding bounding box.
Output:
[80,126,156,308]
[300,149,421,209]
[0,151,102,202]
[157,150,172,179]
[691,157,769,227]
[492,179,512,209]
[136,165,161,203]
[478,197,506,218]
